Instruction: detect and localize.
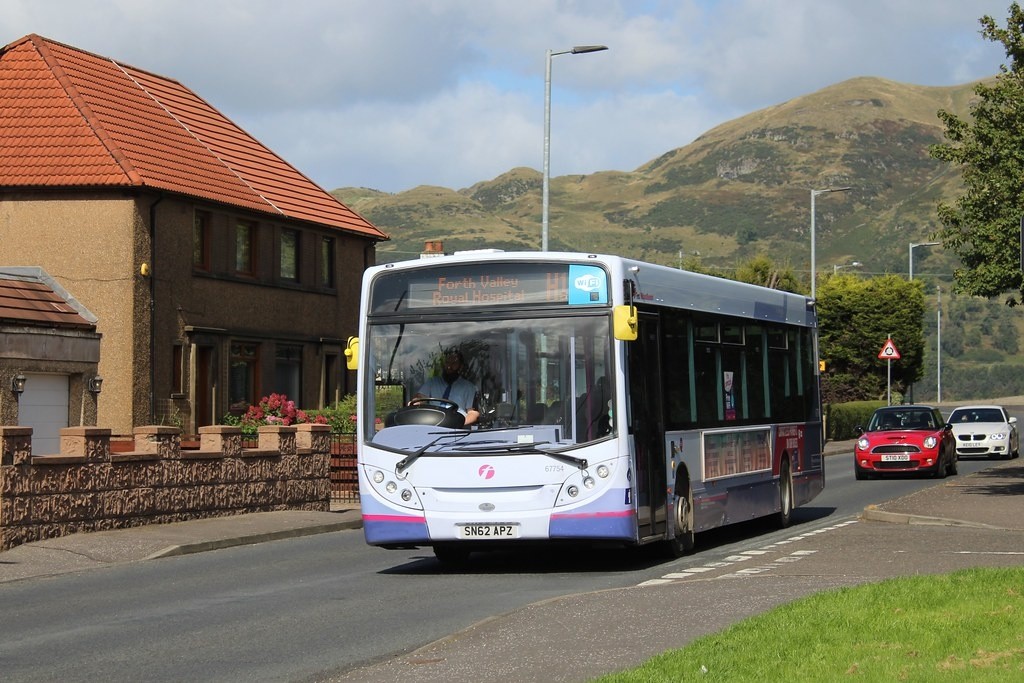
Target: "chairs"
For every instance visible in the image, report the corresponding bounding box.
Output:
[920,413,931,426]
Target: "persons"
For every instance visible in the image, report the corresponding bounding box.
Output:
[407,351,480,432]
[876,414,894,429]
[918,413,933,429]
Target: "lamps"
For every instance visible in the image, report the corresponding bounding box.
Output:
[10,373,27,397]
[89,375,104,394]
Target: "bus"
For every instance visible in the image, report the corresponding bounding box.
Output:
[342,248,826,562]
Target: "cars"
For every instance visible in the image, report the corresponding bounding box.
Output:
[945,404,1020,461]
[854,405,958,481]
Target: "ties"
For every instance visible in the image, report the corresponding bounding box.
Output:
[441,382,453,408]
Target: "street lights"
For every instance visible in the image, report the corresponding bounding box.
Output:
[832,262,865,278]
[908,240,940,282]
[809,184,852,301]
[539,43,612,253]
